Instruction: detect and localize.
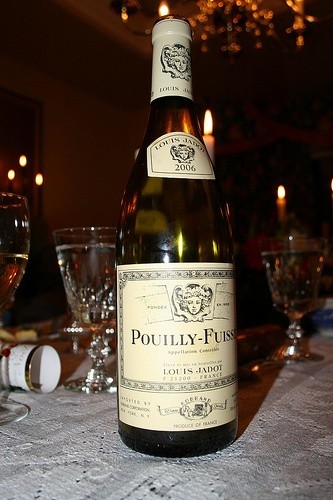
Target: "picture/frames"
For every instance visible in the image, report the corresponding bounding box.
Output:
[0,88,43,230]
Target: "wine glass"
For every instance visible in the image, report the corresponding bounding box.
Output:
[51,226,117,395]
[0,191,32,427]
[260,235,328,364]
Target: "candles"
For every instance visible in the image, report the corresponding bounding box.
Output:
[276,185,286,219]
[201,109,216,170]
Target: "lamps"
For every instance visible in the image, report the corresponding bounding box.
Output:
[110,0,307,57]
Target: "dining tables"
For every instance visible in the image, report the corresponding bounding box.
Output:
[0,297,333,500]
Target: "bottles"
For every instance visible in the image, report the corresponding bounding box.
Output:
[114,15,240,459]
